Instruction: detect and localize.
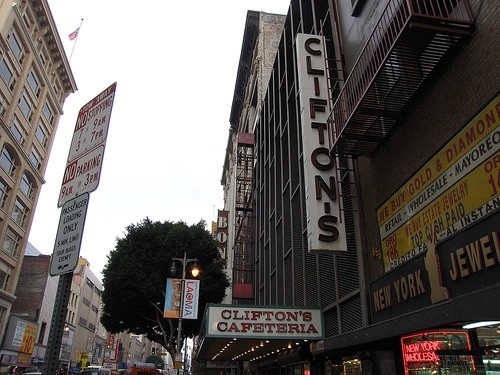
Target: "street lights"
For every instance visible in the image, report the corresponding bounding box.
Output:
[169,251,200,374]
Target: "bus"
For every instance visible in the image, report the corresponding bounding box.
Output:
[82,366,111,375]
